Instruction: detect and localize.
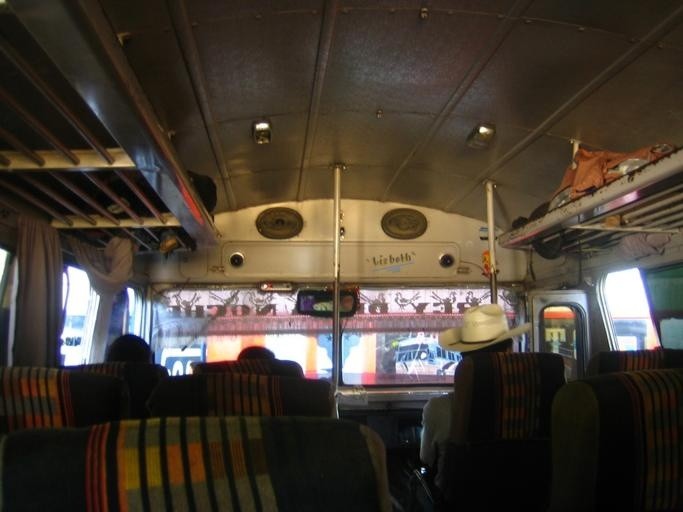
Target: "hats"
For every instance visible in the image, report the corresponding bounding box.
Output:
[438,305,533,352]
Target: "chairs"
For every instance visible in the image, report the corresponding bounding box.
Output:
[412,352,680,508]
[1,350,395,512]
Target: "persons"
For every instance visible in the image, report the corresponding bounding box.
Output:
[340,292,354,312]
[105,334,152,362]
[420,304,532,487]
[238,346,275,359]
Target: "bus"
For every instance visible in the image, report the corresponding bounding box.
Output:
[393,338,462,381]
